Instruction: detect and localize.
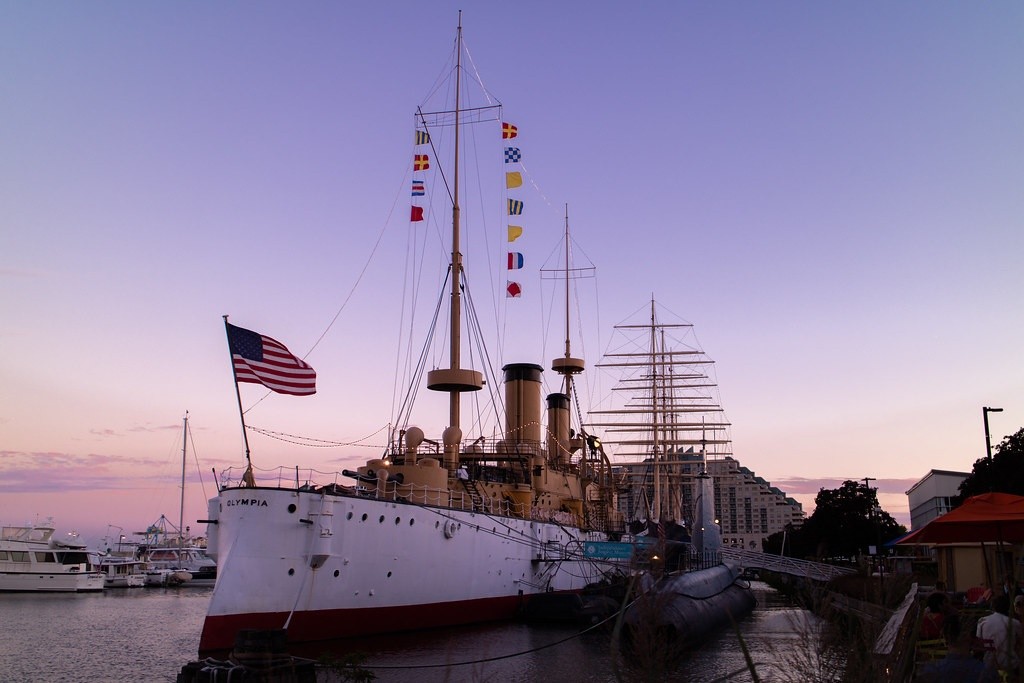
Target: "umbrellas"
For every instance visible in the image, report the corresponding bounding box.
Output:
[896,492,1024,572]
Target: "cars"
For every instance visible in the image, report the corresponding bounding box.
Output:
[919,592,960,639]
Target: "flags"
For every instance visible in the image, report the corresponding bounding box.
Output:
[502,121,518,139]
[506,280,521,299]
[508,225,522,242]
[416,130,430,145]
[503,146,522,163]
[227,322,316,396]
[414,154,430,171]
[507,197,523,215]
[411,206,423,221]
[412,181,425,197]
[506,172,523,190]
[508,252,524,269]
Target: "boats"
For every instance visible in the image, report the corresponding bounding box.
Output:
[91,551,148,587]
[0,526,107,593]
[147,547,217,587]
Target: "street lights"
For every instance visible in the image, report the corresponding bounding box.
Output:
[862,478,876,518]
[118,535,125,552]
[983,407,1003,493]
[868,544,885,608]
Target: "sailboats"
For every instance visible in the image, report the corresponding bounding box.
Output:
[198,10,859,658]
[147,418,192,585]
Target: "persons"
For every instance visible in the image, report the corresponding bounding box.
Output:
[911,575,1024,683]
[926,582,964,643]
